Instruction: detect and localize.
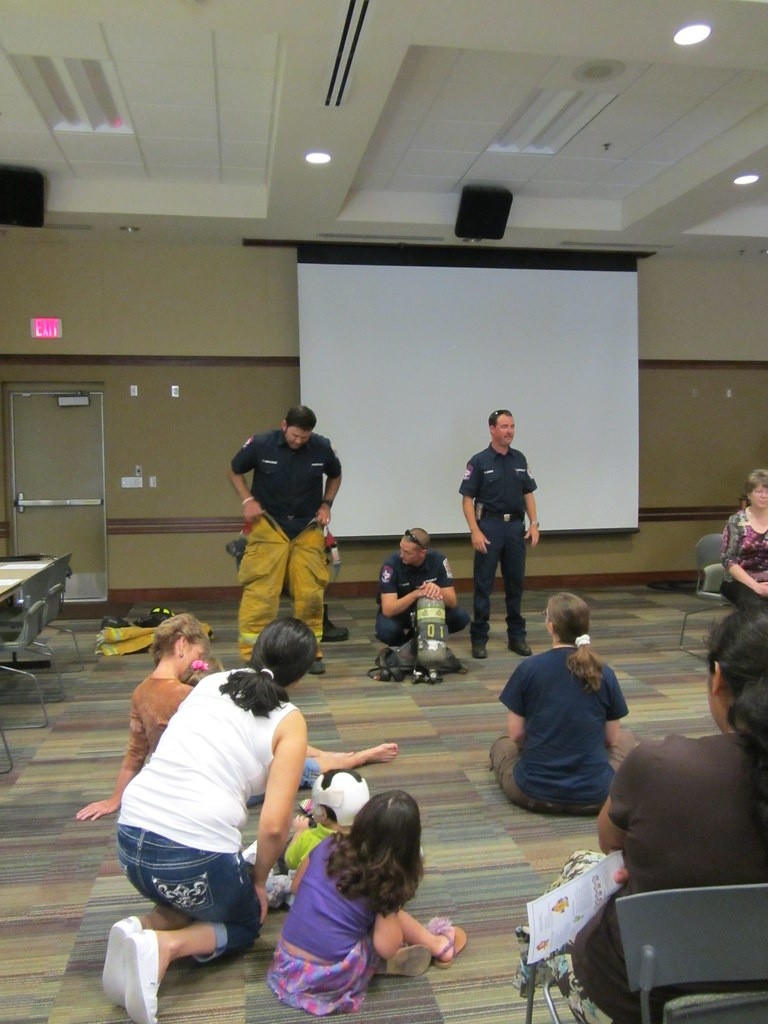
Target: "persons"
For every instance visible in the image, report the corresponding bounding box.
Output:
[720,470,768,612]
[458,410,540,657]
[509,607,768,1024]
[74,615,466,1024]
[490,592,637,816]
[375,528,471,647]
[228,405,342,675]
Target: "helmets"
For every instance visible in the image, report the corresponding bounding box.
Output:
[312,769,370,826]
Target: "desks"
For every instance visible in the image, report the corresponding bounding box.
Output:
[0,556,62,669]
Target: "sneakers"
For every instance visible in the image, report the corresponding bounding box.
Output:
[103,916,159,1024]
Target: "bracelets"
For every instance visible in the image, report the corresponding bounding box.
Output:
[243,496,253,505]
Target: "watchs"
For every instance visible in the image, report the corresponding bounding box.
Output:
[531,521,539,527]
[322,500,333,508]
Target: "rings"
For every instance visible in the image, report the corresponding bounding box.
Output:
[327,519,329,522]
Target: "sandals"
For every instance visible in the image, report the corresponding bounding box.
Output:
[375,945,432,976]
[433,926,468,967]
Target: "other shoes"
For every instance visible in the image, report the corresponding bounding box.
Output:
[321,604,349,642]
[309,659,326,674]
[471,645,487,658]
[508,639,533,656]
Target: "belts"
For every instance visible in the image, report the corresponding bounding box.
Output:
[488,513,522,521]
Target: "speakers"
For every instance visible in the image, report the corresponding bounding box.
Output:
[0,163,44,227]
[455,185,513,239]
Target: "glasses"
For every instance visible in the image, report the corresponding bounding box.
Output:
[405,529,422,546]
[494,410,513,416]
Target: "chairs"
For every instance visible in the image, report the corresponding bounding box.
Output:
[680,533,725,662]
[0,551,74,774]
[526,881,768,1024]
